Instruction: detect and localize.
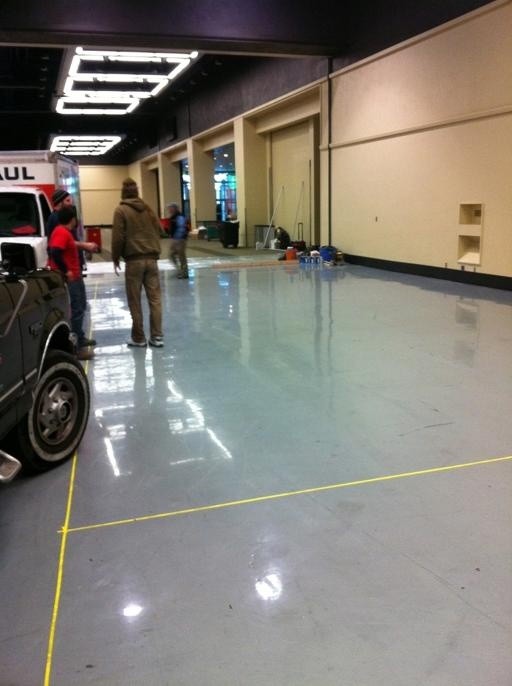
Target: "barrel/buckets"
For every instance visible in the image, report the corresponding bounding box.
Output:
[322,246,337,266]
[286,249,296,260]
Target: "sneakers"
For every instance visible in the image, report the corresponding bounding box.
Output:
[176,271,189,279]
[149,335,163,347]
[125,336,147,347]
[78,337,97,347]
[76,348,96,360]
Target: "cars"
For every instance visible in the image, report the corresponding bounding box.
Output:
[0,255,87,483]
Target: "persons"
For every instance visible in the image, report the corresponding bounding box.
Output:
[110,176,166,347]
[167,202,189,279]
[46,205,95,360]
[224,209,237,221]
[44,188,99,347]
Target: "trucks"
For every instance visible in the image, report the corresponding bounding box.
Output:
[1,148,87,270]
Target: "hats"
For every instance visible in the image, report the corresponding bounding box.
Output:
[166,202,178,207]
[52,189,70,204]
[121,178,139,200]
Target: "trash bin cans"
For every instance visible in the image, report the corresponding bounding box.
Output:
[220,221,239,248]
[255,226,275,248]
